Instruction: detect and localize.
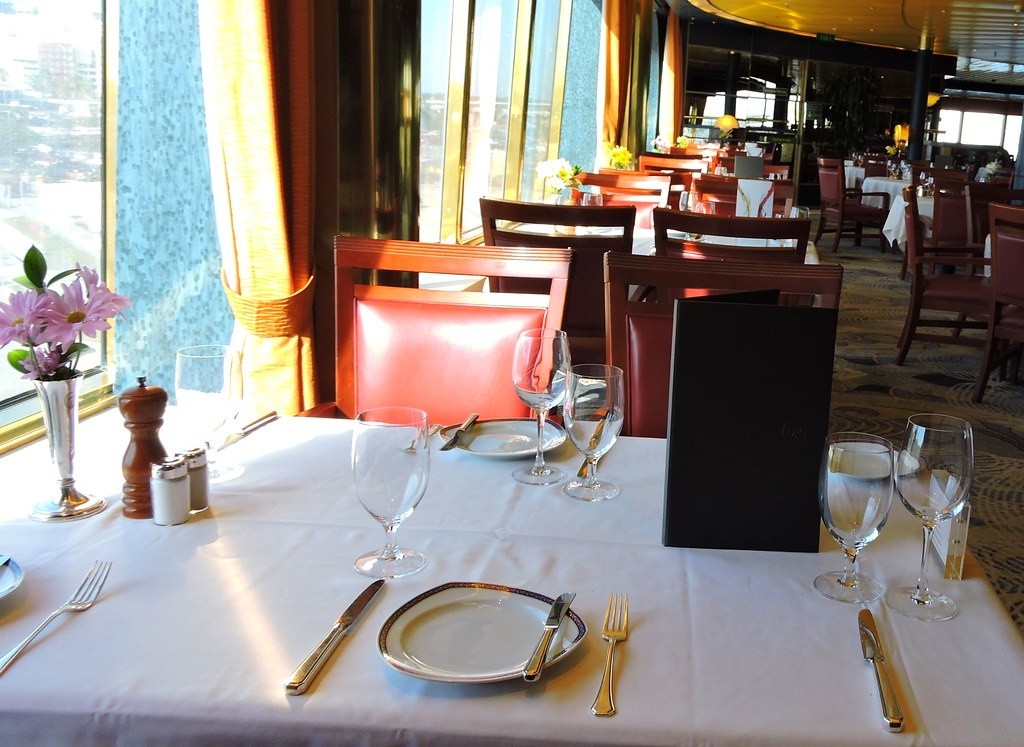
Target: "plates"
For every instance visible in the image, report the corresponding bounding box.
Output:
[439,416,567,460]
[377,581,588,683]
[0,556,25,598]
[827,442,920,477]
[438,417,567,461]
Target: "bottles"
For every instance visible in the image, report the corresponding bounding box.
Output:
[148,446,212,527]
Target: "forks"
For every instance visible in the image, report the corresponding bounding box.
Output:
[591,592,630,717]
[0,559,113,677]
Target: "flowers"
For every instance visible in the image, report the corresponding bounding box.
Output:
[607,145,633,171]
[0,244,132,382]
[676,135,689,149]
[536,156,584,194]
[650,134,671,154]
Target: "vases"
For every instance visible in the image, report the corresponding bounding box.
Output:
[550,195,561,205]
[28,374,108,522]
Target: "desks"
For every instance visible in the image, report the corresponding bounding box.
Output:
[881,194,936,281]
[855,176,912,247]
[1,404,1024,747]
[844,167,865,189]
[511,222,819,266]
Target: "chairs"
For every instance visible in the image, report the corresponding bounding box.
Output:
[284,144,1024,440]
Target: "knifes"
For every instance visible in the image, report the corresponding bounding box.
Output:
[523,592,578,683]
[858,608,906,733]
[282,578,386,695]
[439,412,479,451]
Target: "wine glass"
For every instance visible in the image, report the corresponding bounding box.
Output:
[882,414,976,624]
[887,160,911,181]
[511,328,571,486]
[852,152,865,168]
[350,406,431,581]
[562,363,624,502]
[174,345,247,485]
[919,172,935,197]
[813,430,895,605]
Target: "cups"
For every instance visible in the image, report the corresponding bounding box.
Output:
[714,166,728,176]
[678,190,716,214]
[583,192,603,207]
